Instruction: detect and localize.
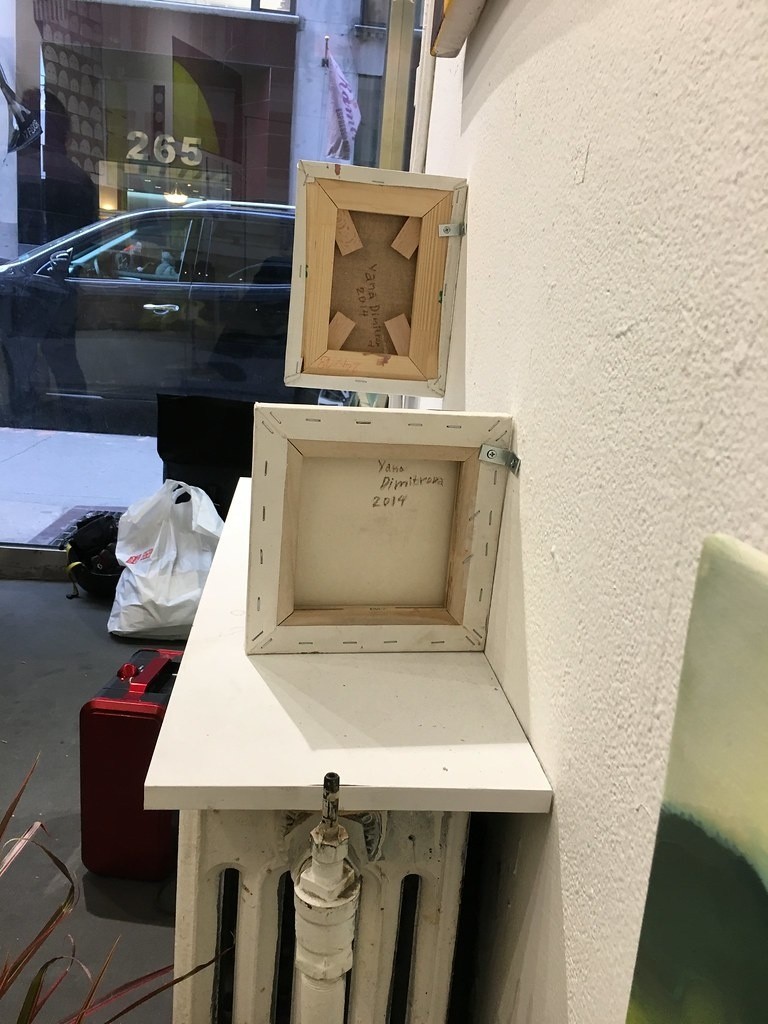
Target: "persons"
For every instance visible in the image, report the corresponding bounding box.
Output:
[0,87,101,433]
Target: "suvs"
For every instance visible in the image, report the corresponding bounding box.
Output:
[0,199,298,429]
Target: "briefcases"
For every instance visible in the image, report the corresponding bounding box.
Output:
[80,648,183,881]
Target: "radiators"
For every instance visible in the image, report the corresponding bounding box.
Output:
[173,805,468,1024]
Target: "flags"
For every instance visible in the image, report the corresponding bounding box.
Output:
[326,50,361,160]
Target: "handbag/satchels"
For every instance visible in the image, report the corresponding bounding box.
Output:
[108,479,225,640]
[157,394,255,522]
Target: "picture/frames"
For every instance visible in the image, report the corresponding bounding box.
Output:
[245,402,513,653]
[284,161,469,398]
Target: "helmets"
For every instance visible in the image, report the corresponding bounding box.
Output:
[58,514,125,599]
